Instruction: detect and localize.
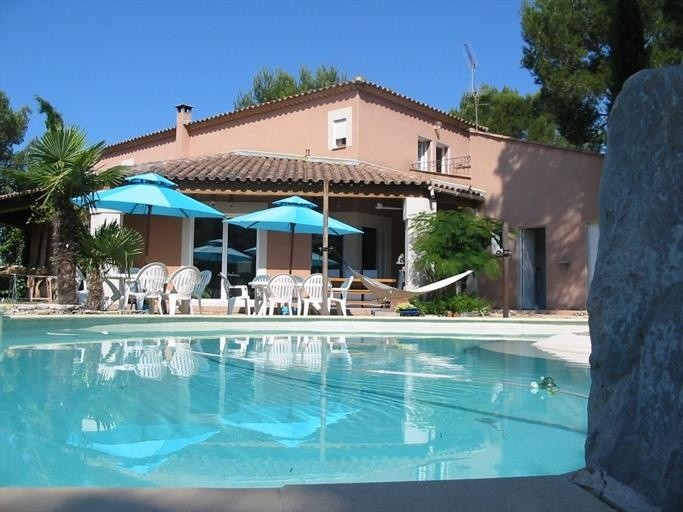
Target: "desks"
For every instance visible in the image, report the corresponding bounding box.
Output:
[101,273,169,310]
[99,340,169,371]
[27,275,54,303]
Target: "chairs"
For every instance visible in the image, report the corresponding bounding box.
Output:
[219,272,250,315]
[229,337,352,370]
[75,266,89,304]
[119,262,211,315]
[74,347,85,375]
[134,338,209,378]
[253,267,354,315]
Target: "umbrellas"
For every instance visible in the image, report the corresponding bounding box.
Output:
[311,252,339,267]
[63,423,221,475]
[222,196,364,315]
[70,170,225,310]
[195,240,253,264]
[245,247,257,254]
[216,404,362,448]
